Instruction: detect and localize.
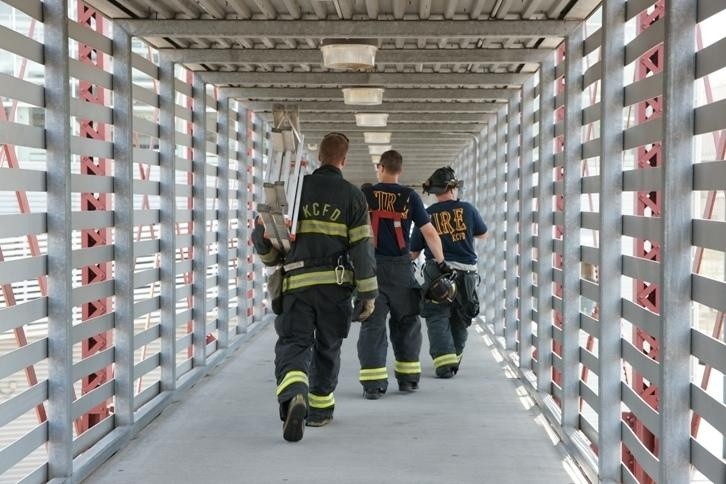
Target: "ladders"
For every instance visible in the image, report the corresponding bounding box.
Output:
[257,102,307,254]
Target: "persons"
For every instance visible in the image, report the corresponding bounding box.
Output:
[353,150,450,403]
[361,183,372,189]
[249,132,377,443]
[409,167,487,378]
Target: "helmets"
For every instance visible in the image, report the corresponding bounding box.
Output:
[421,273,459,317]
[423,167,463,193]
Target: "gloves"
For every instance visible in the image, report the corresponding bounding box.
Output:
[359,298,376,322]
[435,261,456,280]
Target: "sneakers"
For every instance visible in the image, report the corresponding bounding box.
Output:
[437,367,457,378]
[305,417,333,426]
[283,394,307,442]
[365,389,385,399]
[398,380,417,391]
